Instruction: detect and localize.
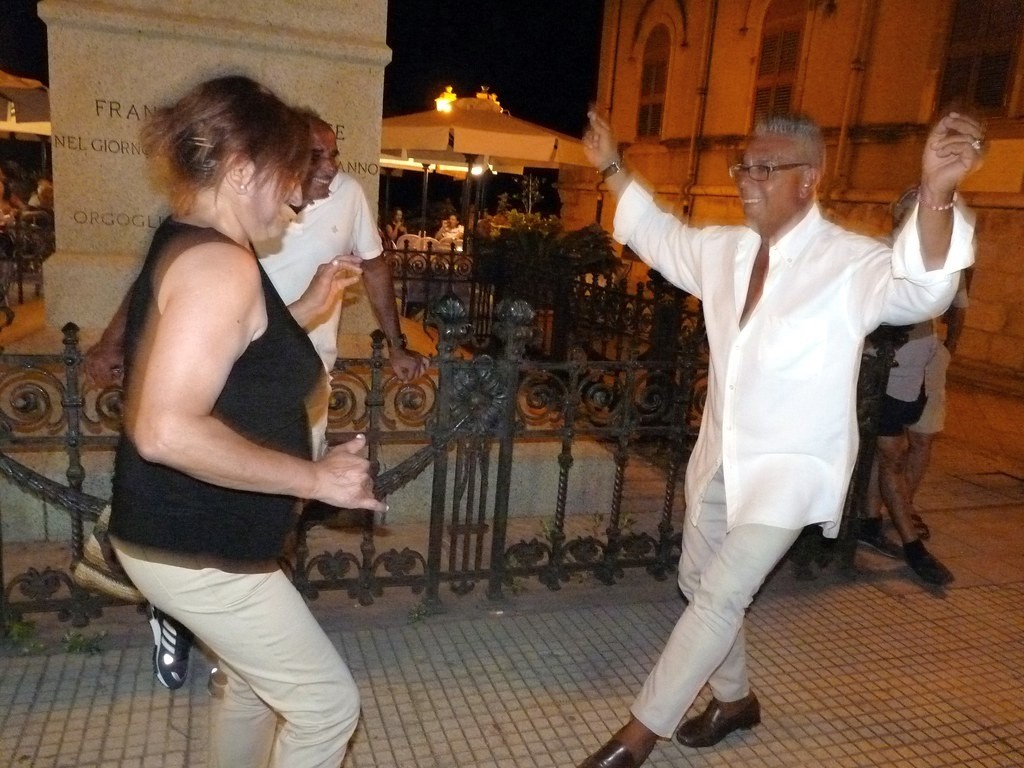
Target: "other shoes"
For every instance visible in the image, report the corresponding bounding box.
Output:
[856,515,905,559]
[904,539,954,585]
[910,514,930,539]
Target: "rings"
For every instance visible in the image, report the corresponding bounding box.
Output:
[972,138,983,150]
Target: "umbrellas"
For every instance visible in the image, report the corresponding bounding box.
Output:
[0,72,52,143]
[380,97,595,251]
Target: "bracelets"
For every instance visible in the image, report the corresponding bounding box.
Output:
[387,334,409,348]
[597,159,625,181]
[916,188,959,212]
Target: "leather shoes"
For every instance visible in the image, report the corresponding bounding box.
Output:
[676,688,761,748]
[576,735,657,768]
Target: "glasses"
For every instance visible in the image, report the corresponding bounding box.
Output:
[729,163,812,183]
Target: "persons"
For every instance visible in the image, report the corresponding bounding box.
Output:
[385,208,465,246]
[578,110,987,768]
[0,168,57,260]
[74,73,431,768]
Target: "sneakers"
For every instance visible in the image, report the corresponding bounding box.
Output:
[207,667,228,697]
[145,600,194,690]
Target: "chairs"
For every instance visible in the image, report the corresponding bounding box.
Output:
[377,225,462,303]
